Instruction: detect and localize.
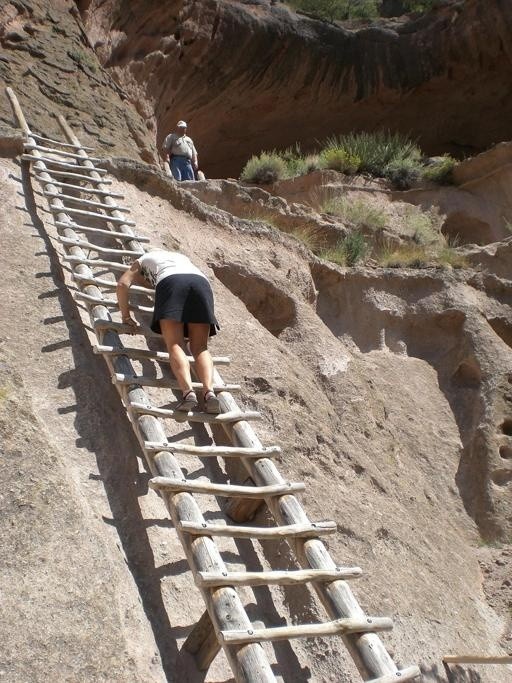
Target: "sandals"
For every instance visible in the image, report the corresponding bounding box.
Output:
[175,389,198,412]
[204,390,222,415]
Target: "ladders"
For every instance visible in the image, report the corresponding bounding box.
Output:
[5,87,422,683]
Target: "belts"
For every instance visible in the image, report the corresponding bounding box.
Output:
[171,155,192,161]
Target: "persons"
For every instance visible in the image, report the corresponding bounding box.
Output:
[194,166,207,180]
[116,248,222,413]
[162,119,198,181]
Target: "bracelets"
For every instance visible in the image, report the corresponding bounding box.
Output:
[123,317,129,320]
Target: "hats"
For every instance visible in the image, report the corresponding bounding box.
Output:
[177,120,187,128]
